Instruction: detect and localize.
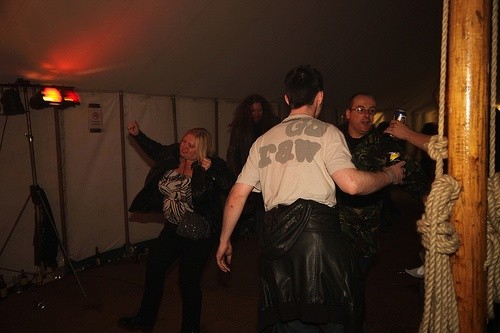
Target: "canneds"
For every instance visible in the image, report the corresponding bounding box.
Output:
[386,151,400,167]
[390,110,407,137]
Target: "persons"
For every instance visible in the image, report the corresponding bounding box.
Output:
[378,78,500,278]
[215,64,406,333]
[117,120,235,333]
[335,92,405,266]
[227,94,283,240]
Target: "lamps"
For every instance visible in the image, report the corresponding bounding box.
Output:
[0,86,81,116]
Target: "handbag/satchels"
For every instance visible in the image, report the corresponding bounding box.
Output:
[177,212,211,240]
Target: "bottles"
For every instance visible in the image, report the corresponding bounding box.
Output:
[0,274,7,300]
[19,269,27,286]
[95,247,102,267]
[36,299,47,312]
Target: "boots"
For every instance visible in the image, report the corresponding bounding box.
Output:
[178,274,202,333]
[118,278,164,333]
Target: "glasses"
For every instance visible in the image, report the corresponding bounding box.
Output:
[352,107,377,115]
